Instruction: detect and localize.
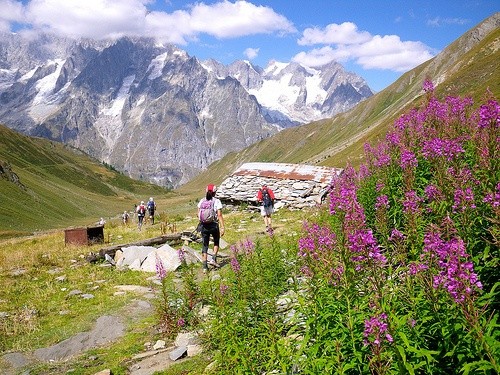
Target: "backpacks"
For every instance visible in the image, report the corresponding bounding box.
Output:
[139,205,144,214]
[199,197,218,224]
[148,201,154,210]
[259,188,273,208]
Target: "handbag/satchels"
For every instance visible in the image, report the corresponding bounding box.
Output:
[197,224,204,232]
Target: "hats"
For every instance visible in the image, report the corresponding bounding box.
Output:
[140,201,144,205]
[149,197,154,201]
[206,184,217,193]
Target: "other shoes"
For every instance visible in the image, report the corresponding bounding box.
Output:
[202,267,210,273]
[209,259,221,267]
[266,227,274,232]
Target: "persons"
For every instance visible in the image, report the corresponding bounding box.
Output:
[197,184,225,273]
[147,197,156,225]
[136,201,147,231]
[257,182,275,228]
[121,211,130,225]
[99,217,107,228]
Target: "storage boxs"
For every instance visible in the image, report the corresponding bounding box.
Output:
[64,225,105,246]
